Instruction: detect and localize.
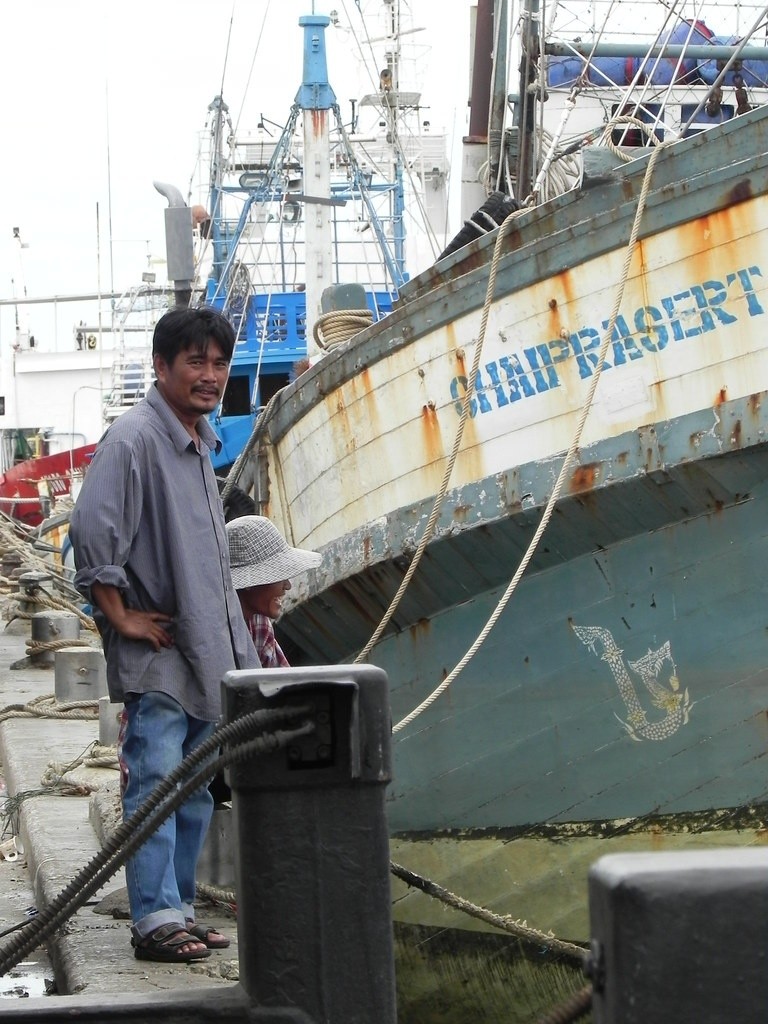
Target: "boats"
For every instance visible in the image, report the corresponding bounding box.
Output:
[0,0,768,1024]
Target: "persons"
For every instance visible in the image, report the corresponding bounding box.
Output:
[117,516,322,810]
[68,310,261,961]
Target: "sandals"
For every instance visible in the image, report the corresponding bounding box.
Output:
[185,915,231,948]
[135,922,212,959]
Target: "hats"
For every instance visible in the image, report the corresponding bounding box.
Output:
[225,515,323,590]
